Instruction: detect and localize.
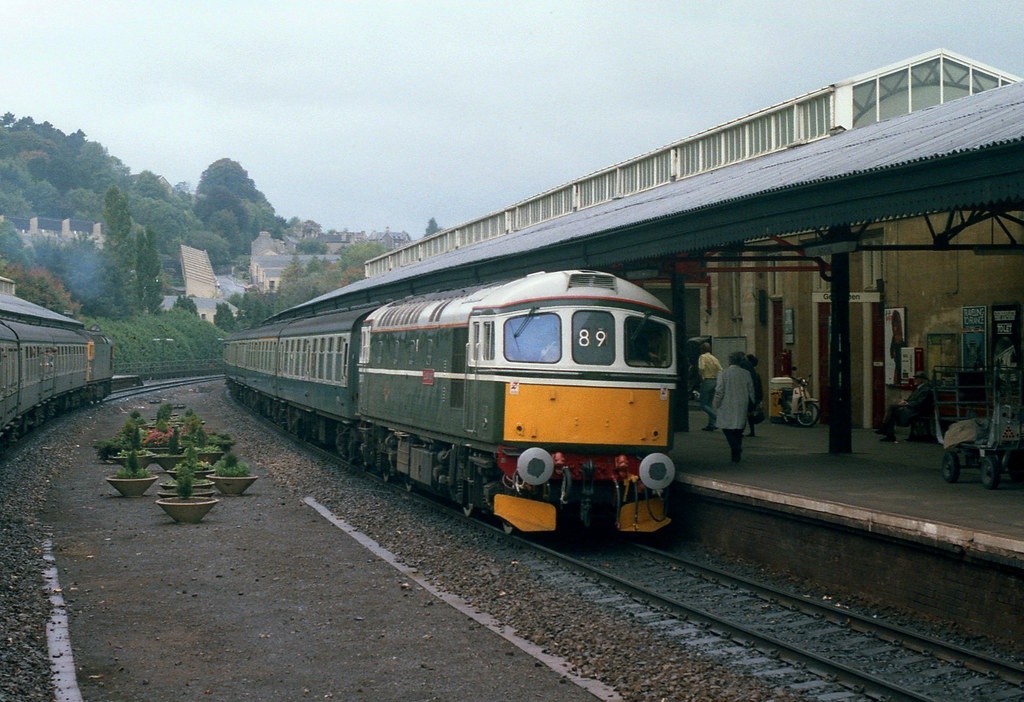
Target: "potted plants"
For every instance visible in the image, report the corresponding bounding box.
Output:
[93,402,260,523]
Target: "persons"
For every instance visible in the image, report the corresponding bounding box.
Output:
[695,342,722,432]
[888,309,904,383]
[742,354,762,437]
[875,372,933,444]
[711,353,754,462]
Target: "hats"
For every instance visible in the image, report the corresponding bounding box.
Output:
[912,371,927,379]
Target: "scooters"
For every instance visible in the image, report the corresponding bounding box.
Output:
[776,358,821,428]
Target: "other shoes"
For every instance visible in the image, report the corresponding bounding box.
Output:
[731,448,742,463]
[702,426,714,431]
[875,428,897,442]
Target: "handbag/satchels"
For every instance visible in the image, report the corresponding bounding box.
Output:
[750,409,764,424]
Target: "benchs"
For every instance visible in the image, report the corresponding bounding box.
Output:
[904,415,970,443]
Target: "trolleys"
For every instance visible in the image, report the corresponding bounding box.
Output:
[928,362,1024,490]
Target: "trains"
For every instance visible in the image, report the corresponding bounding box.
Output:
[222,270,677,541]
[0,294,113,457]
